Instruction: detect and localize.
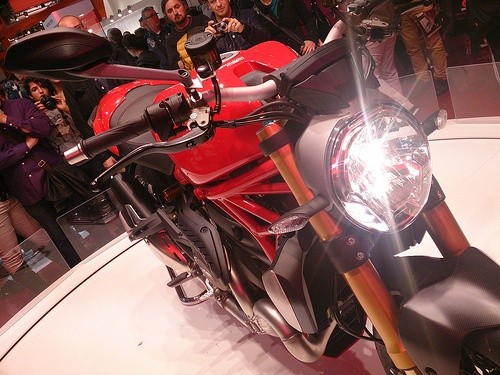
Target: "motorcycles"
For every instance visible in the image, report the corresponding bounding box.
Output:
[0,0,500,375]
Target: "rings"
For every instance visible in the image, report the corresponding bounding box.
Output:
[232,22,237,26]
[310,49,312,51]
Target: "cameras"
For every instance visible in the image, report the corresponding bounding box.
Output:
[212,21,228,34]
[39,95,58,111]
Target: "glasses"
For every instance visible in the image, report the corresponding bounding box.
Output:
[145,13,159,21]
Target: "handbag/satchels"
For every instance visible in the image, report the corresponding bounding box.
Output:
[42,164,99,207]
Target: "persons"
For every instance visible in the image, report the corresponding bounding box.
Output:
[0,0,500,293]
